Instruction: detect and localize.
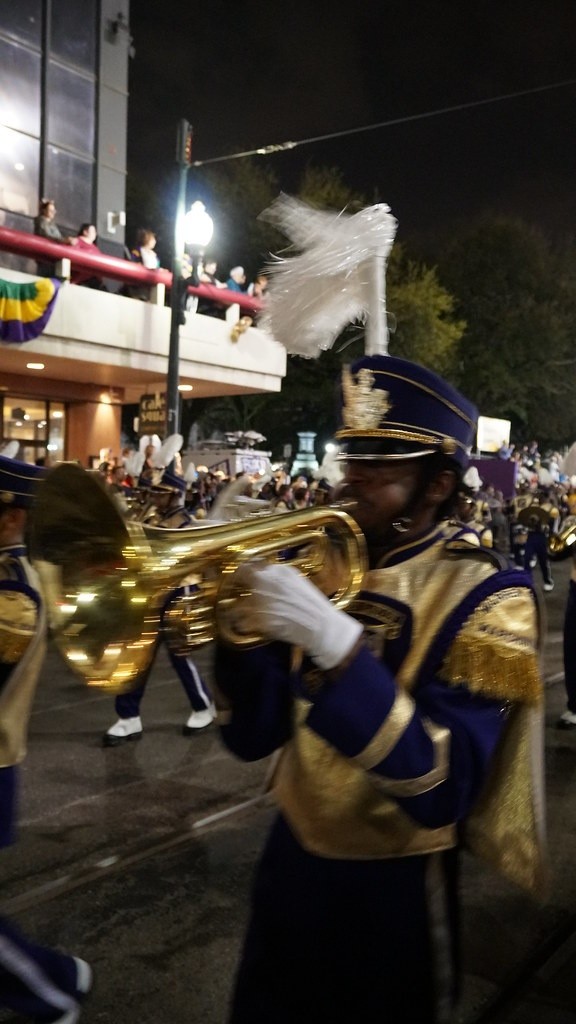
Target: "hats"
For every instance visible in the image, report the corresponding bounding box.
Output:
[259,194,478,468]
[147,434,187,498]
[0,456,52,507]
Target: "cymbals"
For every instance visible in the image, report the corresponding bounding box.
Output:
[518,506,550,528]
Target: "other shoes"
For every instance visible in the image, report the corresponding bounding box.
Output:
[543,579,554,591]
[187,706,215,728]
[54,958,92,1024]
[560,711,576,723]
[106,716,142,737]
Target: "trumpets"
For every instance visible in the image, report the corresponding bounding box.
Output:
[20,459,374,698]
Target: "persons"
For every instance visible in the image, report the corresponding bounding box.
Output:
[34,198,271,329]
[0,452,95,1024]
[212,356,539,1024]
[99,440,576,746]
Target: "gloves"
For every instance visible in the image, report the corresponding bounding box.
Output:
[232,564,364,670]
